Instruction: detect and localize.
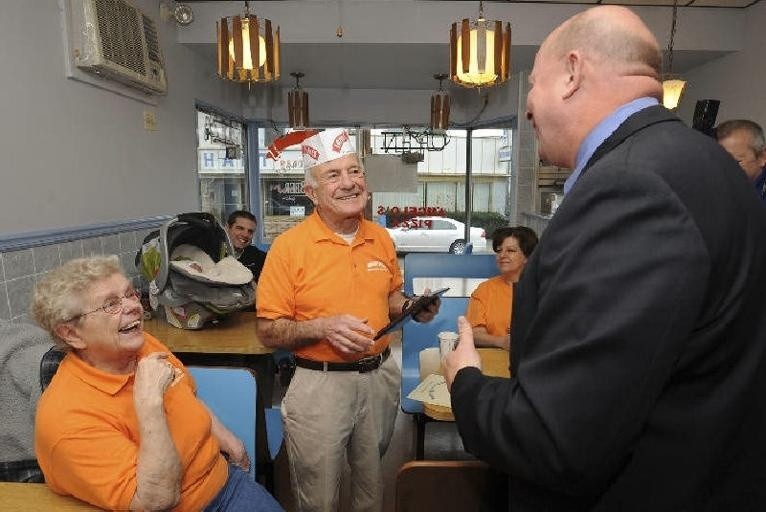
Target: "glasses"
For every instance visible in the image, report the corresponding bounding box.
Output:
[62,288,142,325]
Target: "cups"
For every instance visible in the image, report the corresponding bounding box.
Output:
[437,329,459,365]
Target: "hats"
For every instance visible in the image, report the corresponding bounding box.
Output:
[301,128,357,171]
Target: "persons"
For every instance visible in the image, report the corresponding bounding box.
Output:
[440,4,765,511]
[253,126,437,510]
[463,227,539,354]
[226,209,266,280]
[32,253,286,510]
[710,118,766,199]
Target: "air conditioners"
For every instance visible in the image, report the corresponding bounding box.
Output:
[59,0,169,101]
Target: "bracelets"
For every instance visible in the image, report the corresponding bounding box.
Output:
[400,293,415,311]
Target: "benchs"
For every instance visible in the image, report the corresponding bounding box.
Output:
[397,251,508,458]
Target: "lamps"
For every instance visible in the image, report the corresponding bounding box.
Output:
[656,0,687,112]
[217,2,278,91]
[428,72,450,132]
[448,2,515,95]
[157,1,193,26]
[287,72,310,130]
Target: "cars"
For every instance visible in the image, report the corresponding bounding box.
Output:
[383,214,488,255]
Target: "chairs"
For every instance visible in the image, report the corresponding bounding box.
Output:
[460,243,475,256]
[245,371,287,495]
[392,457,514,510]
[160,364,262,510]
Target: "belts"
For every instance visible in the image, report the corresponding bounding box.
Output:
[295,343,392,374]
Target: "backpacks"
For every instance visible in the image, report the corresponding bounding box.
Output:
[132,212,258,332]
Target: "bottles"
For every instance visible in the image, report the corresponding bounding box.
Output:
[218,241,229,260]
